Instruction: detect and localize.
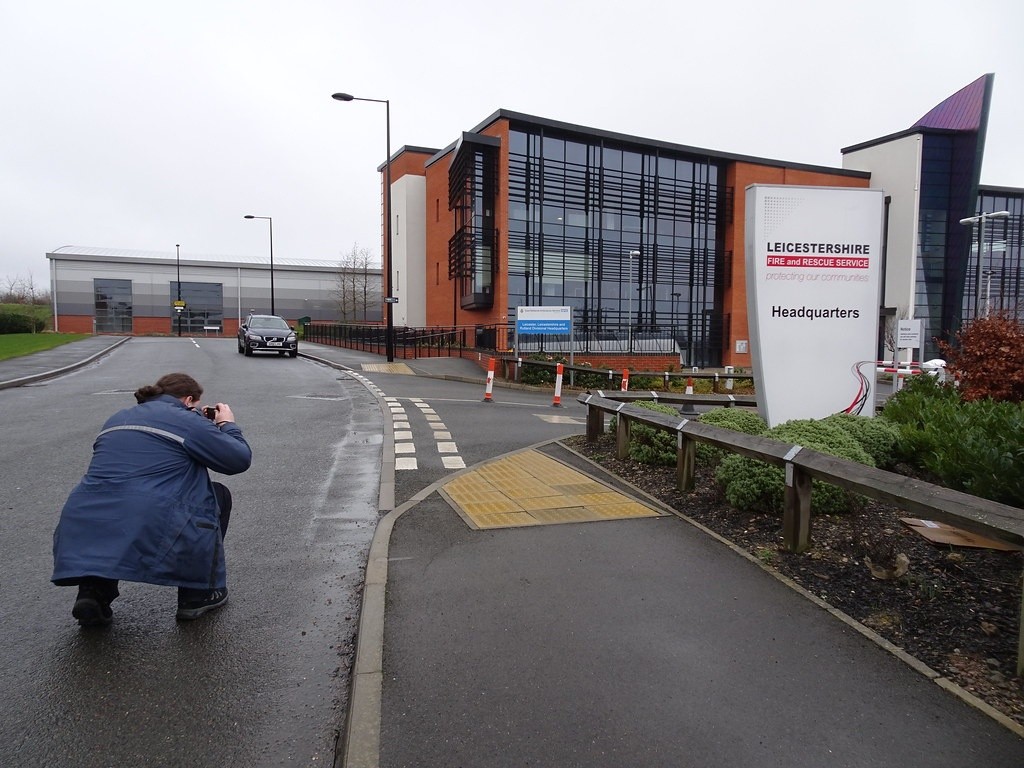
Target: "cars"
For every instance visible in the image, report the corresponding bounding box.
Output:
[236,313,296,356]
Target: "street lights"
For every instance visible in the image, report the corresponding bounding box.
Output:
[244,215,274,315]
[331,93,394,362]
[636,285,652,311]
[670,292,682,337]
[959,211,1011,319]
[629,250,640,352]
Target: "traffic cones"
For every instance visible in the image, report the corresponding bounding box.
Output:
[678,377,699,415]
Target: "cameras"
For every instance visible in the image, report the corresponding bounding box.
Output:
[207,407,218,419]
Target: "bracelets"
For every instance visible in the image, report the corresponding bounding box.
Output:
[216,421,228,425]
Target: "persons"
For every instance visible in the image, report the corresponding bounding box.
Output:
[52,372,252,624]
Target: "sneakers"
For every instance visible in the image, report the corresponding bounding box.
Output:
[175,587,230,619]
[72,582,113,626]
